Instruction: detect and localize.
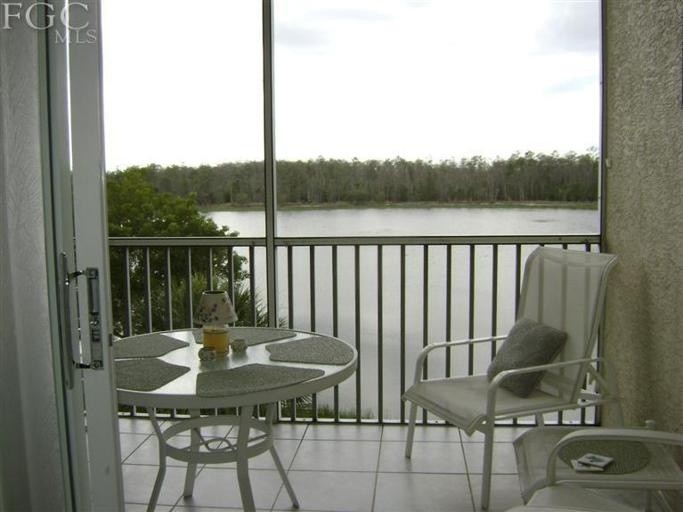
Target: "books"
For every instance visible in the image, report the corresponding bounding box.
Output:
[571,453,613,472]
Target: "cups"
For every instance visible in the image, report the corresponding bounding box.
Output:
[198,346,217,362]
[231,339,247,353]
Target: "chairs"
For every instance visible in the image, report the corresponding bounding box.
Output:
[513,418,683,512]
[402,246,619,510]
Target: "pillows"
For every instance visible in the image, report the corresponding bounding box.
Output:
[488,318,567,398]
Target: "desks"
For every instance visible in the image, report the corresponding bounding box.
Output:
[113,328,358,512]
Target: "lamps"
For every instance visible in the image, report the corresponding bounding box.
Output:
[195,290,238,356]
